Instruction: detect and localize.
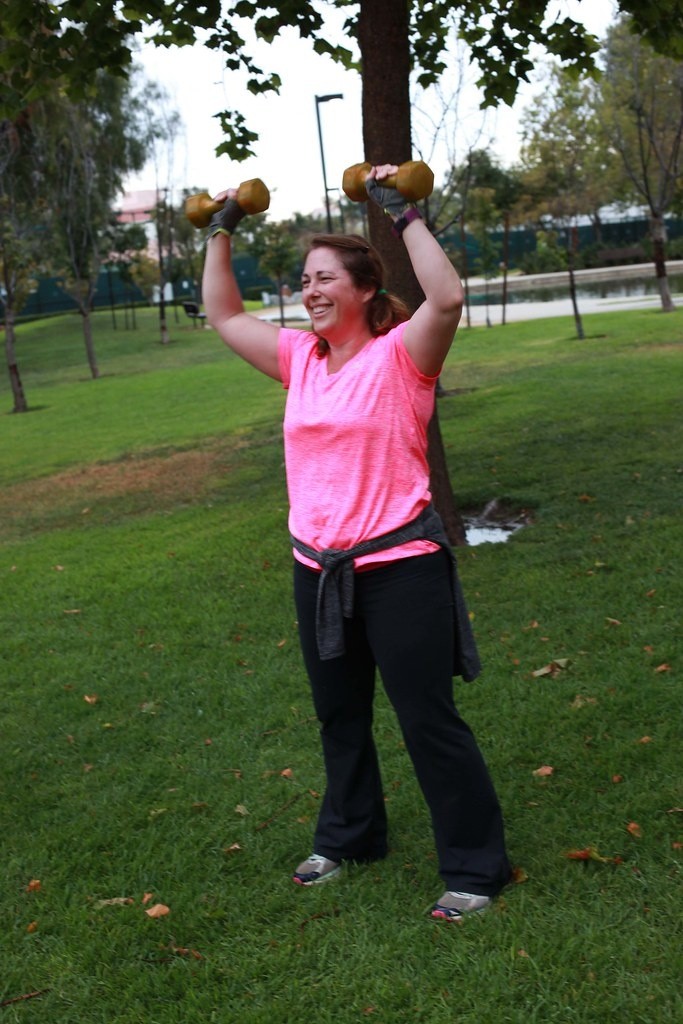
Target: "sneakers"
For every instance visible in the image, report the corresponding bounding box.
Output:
[430,891,490,921]
[292,853,342,884]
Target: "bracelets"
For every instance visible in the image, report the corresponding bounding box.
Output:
[391,208,421,239]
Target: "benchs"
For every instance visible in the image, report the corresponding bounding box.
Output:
[183,301,208,328]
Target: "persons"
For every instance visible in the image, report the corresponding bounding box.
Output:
[203,162,511,921]
